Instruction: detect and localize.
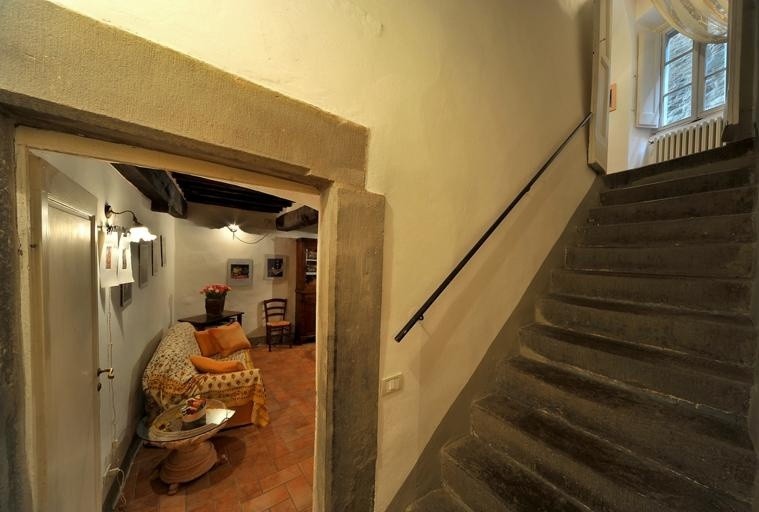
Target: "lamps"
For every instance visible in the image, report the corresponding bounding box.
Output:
[105,204,157,242]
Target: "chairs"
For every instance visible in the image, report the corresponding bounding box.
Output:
[263,298,292,352]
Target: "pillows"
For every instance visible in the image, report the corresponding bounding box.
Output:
[194,329,219,358]
[207,322,252,357]
[190,355,246,374]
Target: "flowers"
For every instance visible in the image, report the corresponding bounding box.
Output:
[200,284,231,298]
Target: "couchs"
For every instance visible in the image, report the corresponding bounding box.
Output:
[140,322,270,432]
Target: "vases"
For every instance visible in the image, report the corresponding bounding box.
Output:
[205,298,224,316]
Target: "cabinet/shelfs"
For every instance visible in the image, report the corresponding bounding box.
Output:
[295,237,317,346]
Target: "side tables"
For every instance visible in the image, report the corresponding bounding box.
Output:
[137,397,237,495]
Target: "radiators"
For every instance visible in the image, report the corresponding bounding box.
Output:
[648,116,723,164]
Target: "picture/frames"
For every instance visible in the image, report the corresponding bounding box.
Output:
[264,254,287,281]
[121,235,167,307]
[227,258,253,289]
[609,83,616,112]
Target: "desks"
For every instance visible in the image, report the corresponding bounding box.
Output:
[178,310,244,331]
